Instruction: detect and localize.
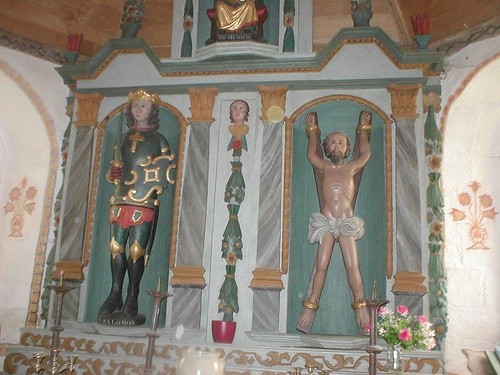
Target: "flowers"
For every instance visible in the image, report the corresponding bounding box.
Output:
[364,305,436,351]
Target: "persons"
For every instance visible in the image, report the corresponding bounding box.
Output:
[227,100,250,156]
[213,0,260,36]
[97,89,171,318]
[297,111,371,329]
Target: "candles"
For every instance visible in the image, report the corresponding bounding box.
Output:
[157,277,160,292]
[59,271,64,287]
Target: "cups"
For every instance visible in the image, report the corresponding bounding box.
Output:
[213,321,236,343]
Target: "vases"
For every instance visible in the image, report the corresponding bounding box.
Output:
[390,350,402,372]
